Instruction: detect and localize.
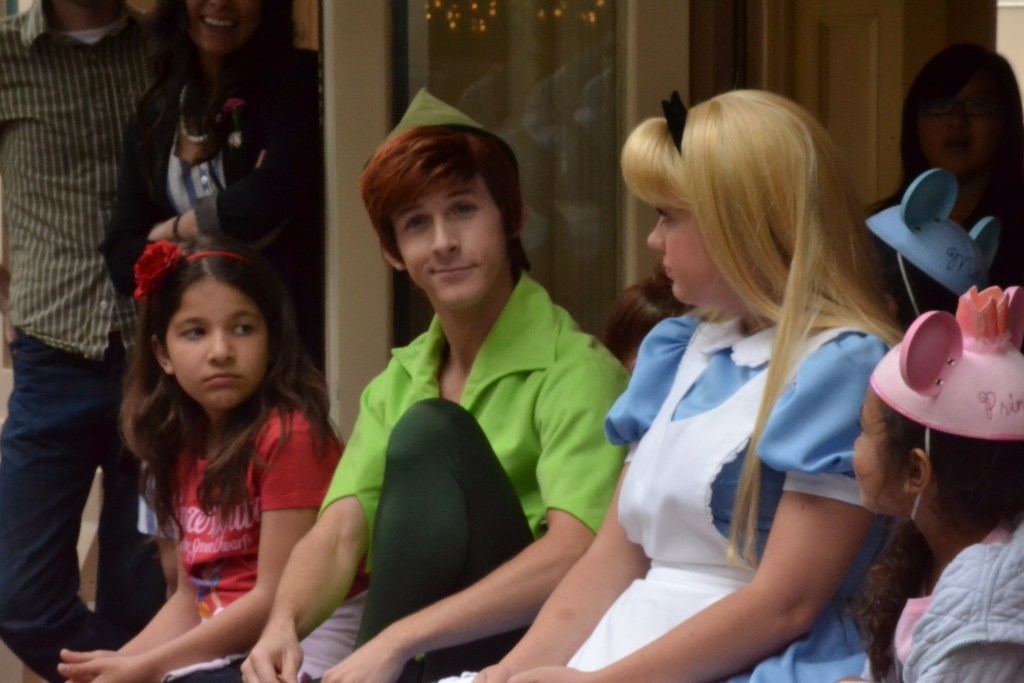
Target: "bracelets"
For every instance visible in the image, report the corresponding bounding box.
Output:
[173,215,182,241]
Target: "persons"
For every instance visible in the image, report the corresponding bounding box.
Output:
[0,0,1024,683]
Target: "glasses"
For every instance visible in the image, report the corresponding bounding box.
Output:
[924,94,1001,116]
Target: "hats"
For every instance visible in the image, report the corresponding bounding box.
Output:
[870,282,1024,442]
[864,167,1001,295]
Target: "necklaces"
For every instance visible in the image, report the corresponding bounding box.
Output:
[180,82,207,142]
